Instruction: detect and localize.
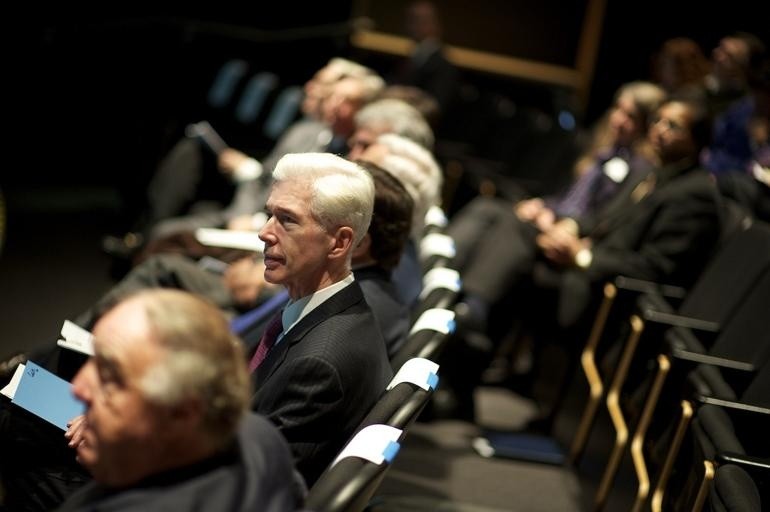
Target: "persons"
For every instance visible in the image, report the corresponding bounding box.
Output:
[415,30,752,426]
[0,0,443,512]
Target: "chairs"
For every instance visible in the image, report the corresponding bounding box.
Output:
[343,358,441,445]
[200,49,307,142]
[392,308,458,373]
[420,231,456,273]
[302,423,405,512]
[424,207,449,236]
[410,266,463,325]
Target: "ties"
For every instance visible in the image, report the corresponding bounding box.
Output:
[247,309,284,376]
